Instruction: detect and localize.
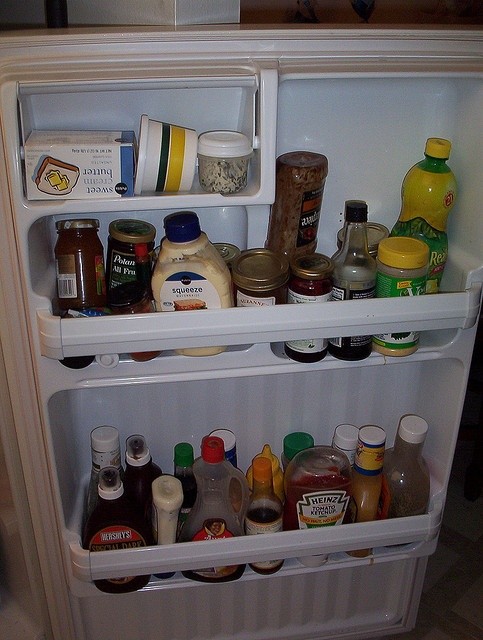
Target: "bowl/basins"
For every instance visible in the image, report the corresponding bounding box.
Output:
[134,114,196,195]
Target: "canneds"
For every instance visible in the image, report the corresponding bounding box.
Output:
[284,255,332,361]
[109,300,162,361]
[55,218,107,309]
[210,239,240,266]
[335,221,390,259]
[232,247,287,306]
[101,219,156,302]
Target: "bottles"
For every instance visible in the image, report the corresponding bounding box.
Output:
[285,447,350,568]
[121,434,161,511]
[85,428,124,514]
[169,442,195,520]
[198,129,254,197]
[282,432,314,463]
[208,428,238,467]
[330,201,377,362]
[181,436,248,581]
[151,474,179,579]
[80,467,152,593]
[393,137,451,293]
[150,211,230,358]
[348,425,387,558]
[247,459,284,574]
[243,442,282,500]
[386,414,431,551]
[332,423,359,466]
